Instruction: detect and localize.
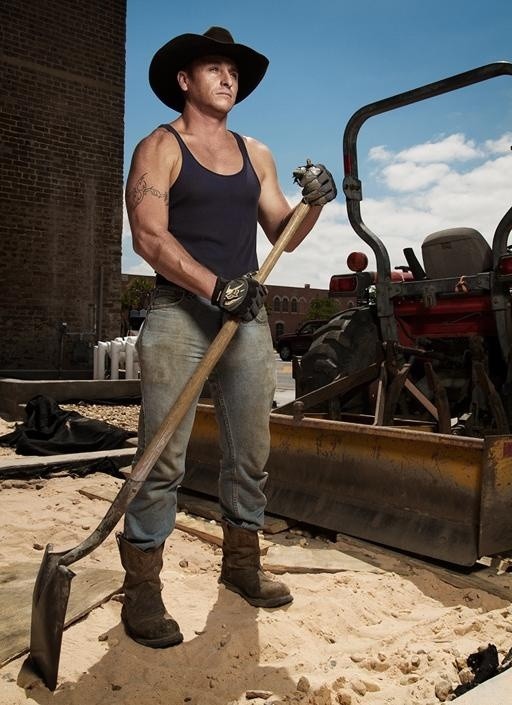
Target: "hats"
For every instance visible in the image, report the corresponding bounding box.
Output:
[149,26,269,113]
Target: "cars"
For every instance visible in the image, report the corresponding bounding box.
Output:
[274,319,328,360]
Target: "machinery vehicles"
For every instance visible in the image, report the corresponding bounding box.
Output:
[182,59,511,567]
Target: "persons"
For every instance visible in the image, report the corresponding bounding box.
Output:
[115,28,337,650]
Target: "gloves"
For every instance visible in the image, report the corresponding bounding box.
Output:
[294,164,336,207]
[212,273,268,322]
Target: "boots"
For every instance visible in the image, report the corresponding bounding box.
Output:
[116,534,183,648]
[219,517,293,608]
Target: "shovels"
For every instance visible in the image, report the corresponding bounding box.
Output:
[29,198,308,690]
